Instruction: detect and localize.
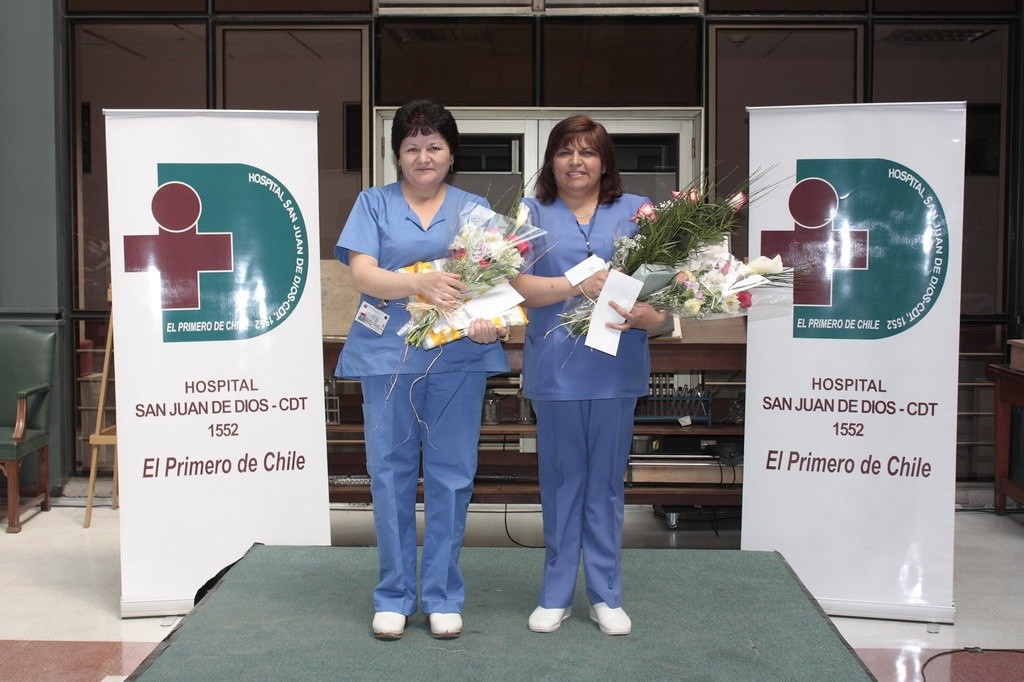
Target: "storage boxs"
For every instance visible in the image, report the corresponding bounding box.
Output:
[630,262,676,301]
[1005,338,1024,371]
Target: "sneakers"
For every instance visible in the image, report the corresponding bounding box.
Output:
[372,611,406,639]
[526,604,572,632]
[588,601,632,636]
[428,613,462,639]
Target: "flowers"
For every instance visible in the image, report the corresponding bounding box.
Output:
[397,166,560,349]
[555,158,826,337]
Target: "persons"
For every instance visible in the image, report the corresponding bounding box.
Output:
[333,99,510,639]
[509,117,667,635]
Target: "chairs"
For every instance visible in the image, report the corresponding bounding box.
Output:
[0,324,57,534]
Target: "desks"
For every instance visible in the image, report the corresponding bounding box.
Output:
[988,362,1024,516]
